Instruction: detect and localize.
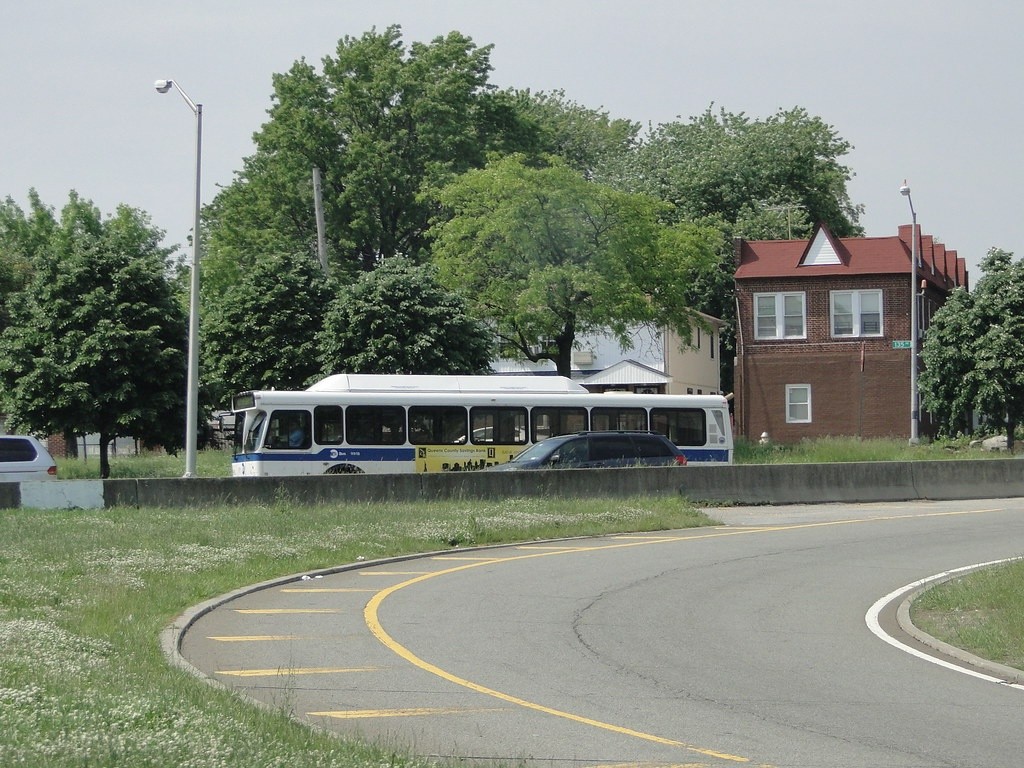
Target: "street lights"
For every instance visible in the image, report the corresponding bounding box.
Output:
[900,177,927,450]
[154,77,203,477]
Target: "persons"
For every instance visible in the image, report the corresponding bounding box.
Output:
[564,441,583,464]
[279,420,304,448]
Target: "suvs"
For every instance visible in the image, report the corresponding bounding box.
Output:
[479,430,687,473]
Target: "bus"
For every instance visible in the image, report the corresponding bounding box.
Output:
[218,374,734,478]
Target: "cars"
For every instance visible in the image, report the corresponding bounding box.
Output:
[0,434,57,482]
